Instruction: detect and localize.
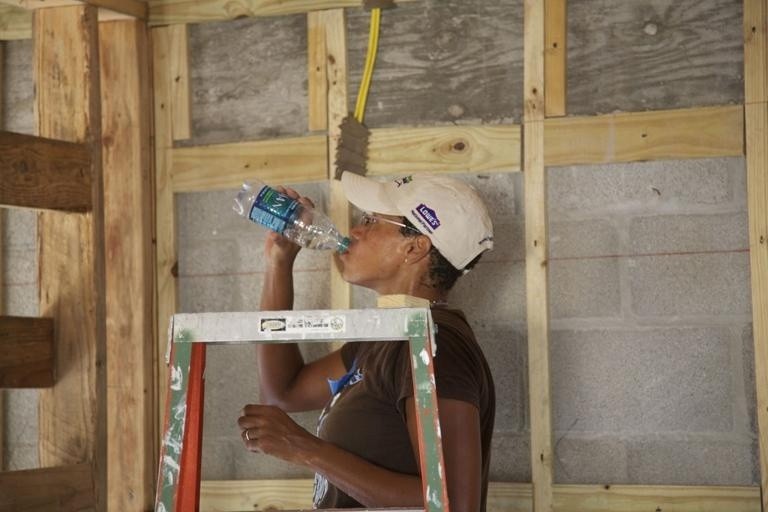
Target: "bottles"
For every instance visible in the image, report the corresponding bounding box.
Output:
[230,176,352,260]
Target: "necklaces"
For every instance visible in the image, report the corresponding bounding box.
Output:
[375,294,452,309]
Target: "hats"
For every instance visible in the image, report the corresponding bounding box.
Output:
[340,171,494,275]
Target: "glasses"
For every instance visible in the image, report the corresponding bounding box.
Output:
[357,211,417,235]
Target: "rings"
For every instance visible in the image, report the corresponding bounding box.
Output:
[246,430,250,441]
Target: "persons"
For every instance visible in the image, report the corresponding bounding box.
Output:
[237,169,494,512]
